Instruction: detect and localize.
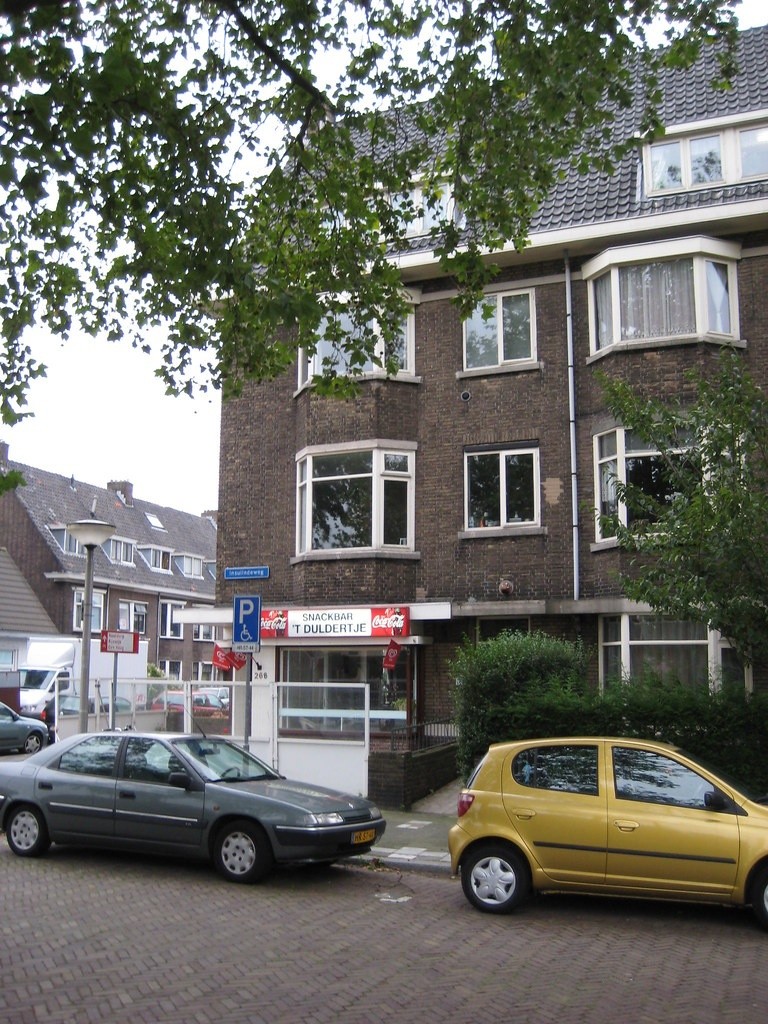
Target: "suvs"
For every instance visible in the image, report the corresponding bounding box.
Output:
[40,692,135,743]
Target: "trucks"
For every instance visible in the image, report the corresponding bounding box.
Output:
[15,635,149,721]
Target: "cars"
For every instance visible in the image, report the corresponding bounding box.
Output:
[448,736,768,930]
[0,702,49,756]
[152,687,230,719]
[0,732,387,883]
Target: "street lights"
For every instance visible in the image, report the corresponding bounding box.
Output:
[65,512,116,733]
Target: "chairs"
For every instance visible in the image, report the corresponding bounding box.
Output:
[125,752,148,779]
[196,698,203,704]
[167,757,186,784]
